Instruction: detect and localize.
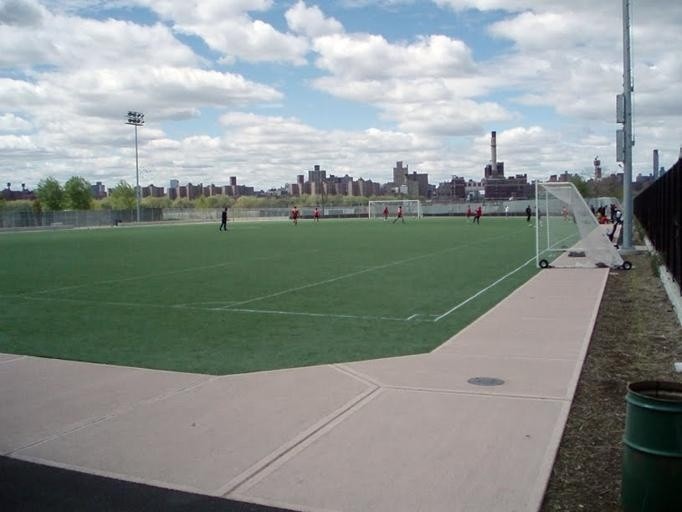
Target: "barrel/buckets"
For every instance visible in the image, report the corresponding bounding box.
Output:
[620,379,682,512]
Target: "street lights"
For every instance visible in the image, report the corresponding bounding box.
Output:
[124,110,145,222]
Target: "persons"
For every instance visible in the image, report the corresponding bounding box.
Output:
[383,207,390,221]
[589,203,618,224]
[534,206,543,228]
[290,205,299,225]
[472,206,482,224]
[220,207,228,232]
[391,205,406,225]
[504,205,509,217]
[465,205,472,221]
[561,205,568,220]
[526,205,533,222]
[313,208,320,223]
